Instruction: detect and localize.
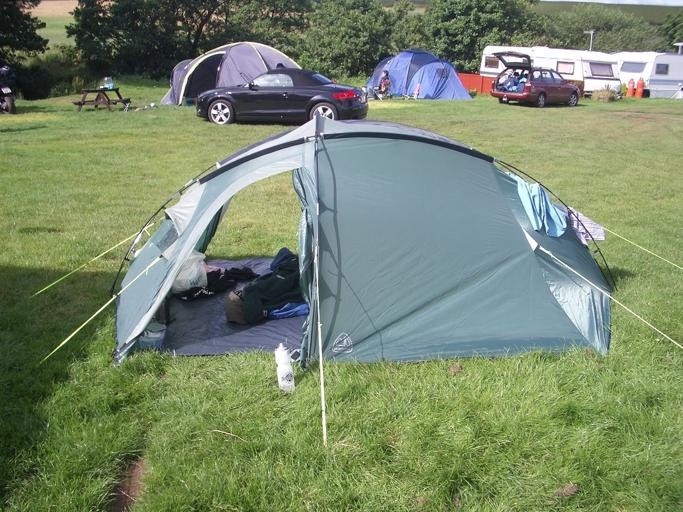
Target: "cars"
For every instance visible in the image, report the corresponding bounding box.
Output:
[491,51,579,108]
[196,68,367,124]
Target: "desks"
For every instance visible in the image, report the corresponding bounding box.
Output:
[77,87,126,111]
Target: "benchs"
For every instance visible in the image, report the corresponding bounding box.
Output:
[73,98,131,113]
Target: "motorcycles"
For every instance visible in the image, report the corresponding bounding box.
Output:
[0,66,16,113]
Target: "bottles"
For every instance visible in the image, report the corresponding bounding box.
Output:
[274,342,295,395]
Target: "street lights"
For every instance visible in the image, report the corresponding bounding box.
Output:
[584,30,595,49]
[672,42,683,54]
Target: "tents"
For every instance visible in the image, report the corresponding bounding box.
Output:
[160,41,302,106]
[112,113,617,364]
[366,50,473,102]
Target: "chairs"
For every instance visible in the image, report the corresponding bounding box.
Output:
[513,72,527,82]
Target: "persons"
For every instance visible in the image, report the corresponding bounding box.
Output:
[372,69,393,100]
